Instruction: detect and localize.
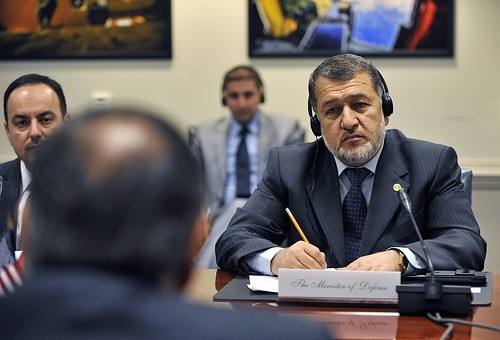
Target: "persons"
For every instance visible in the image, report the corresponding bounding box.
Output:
[189,65,306,271]
[0,73,71,299]
[0,108,331,340]
[214,53,488,278]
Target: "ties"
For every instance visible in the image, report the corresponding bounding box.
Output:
[18,192,32,252]
[342,168,370,266]
[236,128,251,198]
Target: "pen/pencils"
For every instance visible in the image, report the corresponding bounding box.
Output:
[284,208,311,246]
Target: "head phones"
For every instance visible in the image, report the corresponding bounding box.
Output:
[222,67,265,106]
[307,72,393,136]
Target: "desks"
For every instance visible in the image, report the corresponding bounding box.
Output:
[185,269,500,340]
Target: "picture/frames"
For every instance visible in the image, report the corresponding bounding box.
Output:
[248,0,454,58]
[0,0,172,61]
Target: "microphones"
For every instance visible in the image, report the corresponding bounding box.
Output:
[395,188,473,319]
